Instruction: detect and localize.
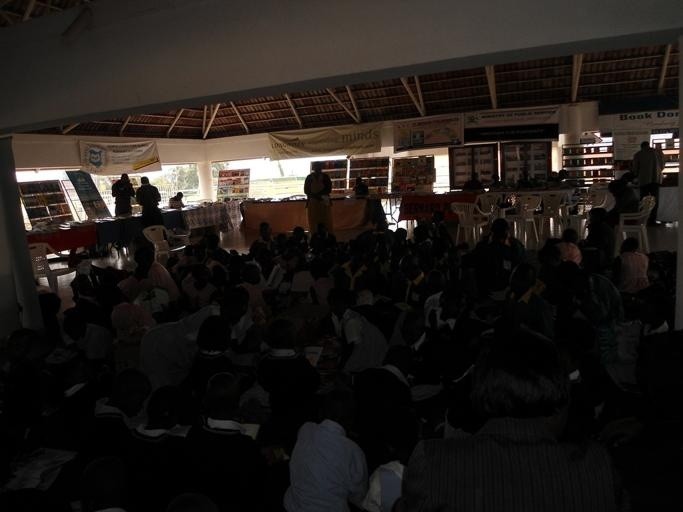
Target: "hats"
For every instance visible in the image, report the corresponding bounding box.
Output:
[560,242,582,264]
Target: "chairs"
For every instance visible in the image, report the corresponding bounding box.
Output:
[451,190,589,243]
[29,242,82,292]
[143,224,191,263]
[614,196,656,254]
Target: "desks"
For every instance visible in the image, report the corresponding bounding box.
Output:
[399,187,575,220]
[27,202,234,254]
[240,195,368,232]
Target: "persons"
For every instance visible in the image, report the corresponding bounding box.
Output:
[463,175,483,190]
[490,174,502,189]
[303,163,335,234]
[111,174,135,216]
[0,207,683,512]
[632,141,665,226]
[135,177,161,214]
[351,177,368,195]
[168,192,184,208]
[604,178,638,228]
